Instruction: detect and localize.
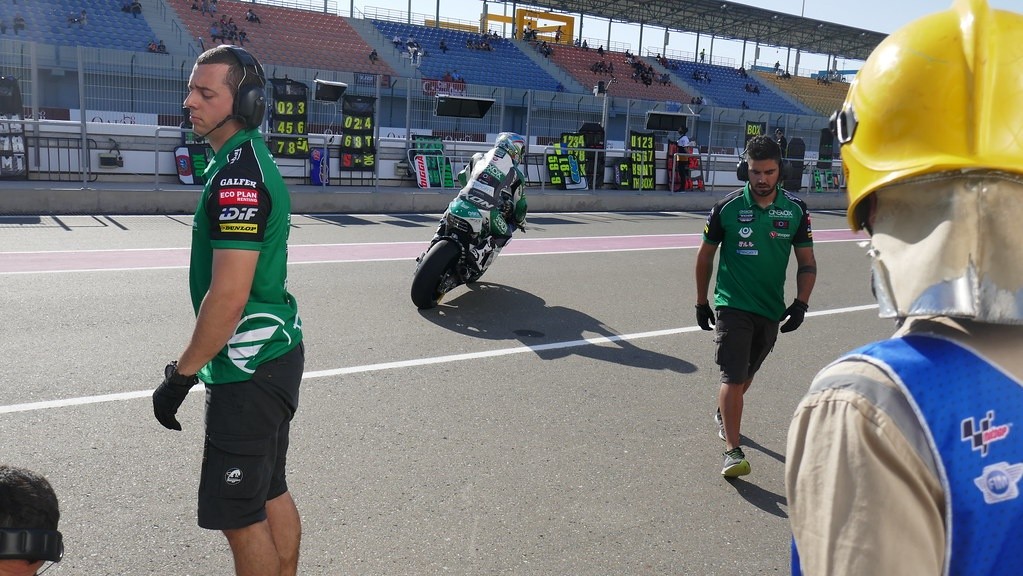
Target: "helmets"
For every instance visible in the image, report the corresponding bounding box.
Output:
[495,129,528,166]
[826,0,1022,234]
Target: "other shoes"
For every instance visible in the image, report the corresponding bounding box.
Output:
[675,186,685,192]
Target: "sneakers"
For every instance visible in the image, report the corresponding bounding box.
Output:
[721,447,752,478]
[714,406,742,442]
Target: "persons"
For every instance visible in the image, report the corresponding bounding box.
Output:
[514,22,712,105]
[68,10,87,28]
[0,13,26,36]
[466,30,499,51]
[816,69,838,85]
[122,0,142,17]
[0,464,60,576]
[148,39,166,53]
[439,39,448,53]
[190,0,260,46]
[412,132,527,274]
[152,45,306,576]
[370,49,377,64]
[776,128,788,189]
[696,135,817,477]
[393,34,424,59]
[444,70,460,82]
[775,61,790,79]
[738,65,760,109]
[785,0,1023,576]
[677,126,689,193]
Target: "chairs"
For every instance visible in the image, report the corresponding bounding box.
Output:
[0,0,852,126]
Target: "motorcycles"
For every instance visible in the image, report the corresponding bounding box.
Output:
[410,215,527,311]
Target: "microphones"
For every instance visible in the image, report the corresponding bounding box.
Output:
[197,115,236,143]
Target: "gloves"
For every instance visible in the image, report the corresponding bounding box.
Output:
[153,361,200,431]
[695,299,716,331]
[779,297,809,333]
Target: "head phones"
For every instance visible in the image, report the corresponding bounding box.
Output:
[735,146,787,182]
[218,45,266,128]
